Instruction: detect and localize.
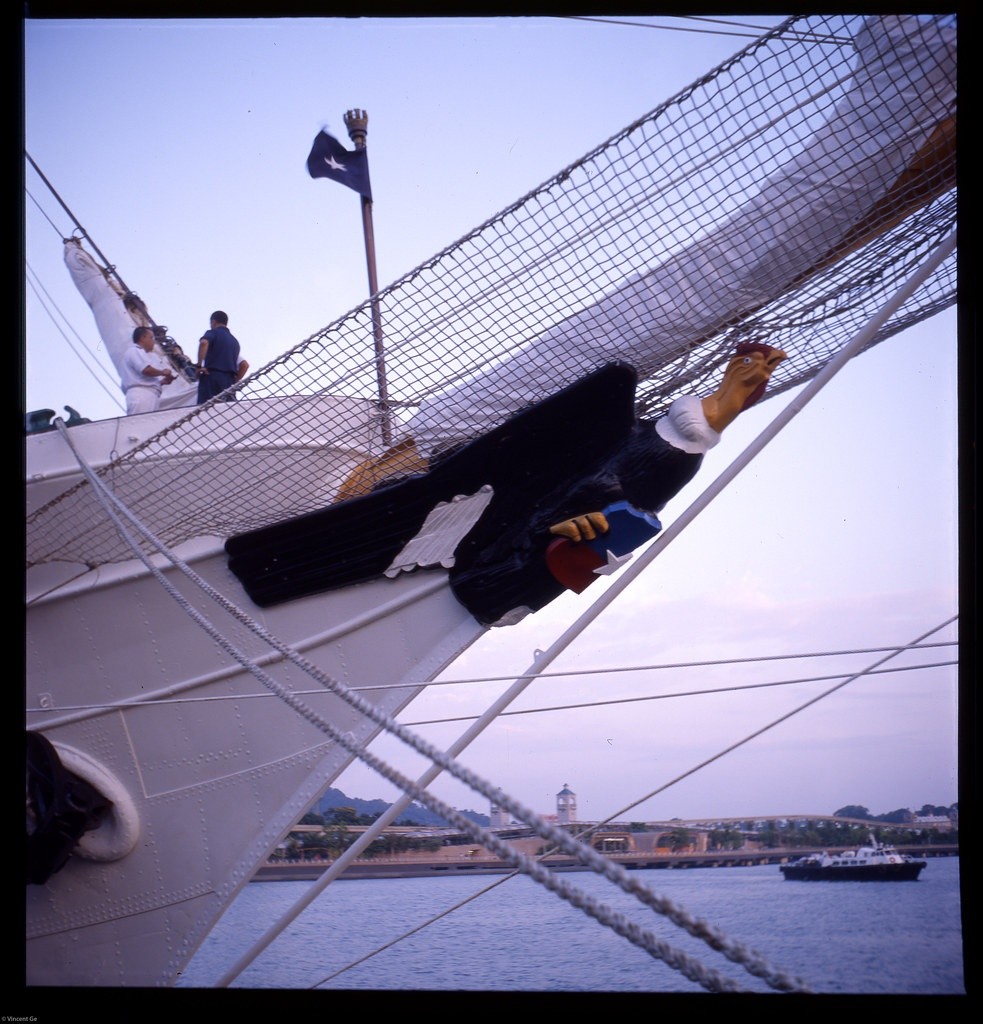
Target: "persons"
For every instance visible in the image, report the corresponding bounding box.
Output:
[120,326,179,416]
[194,310,249,407]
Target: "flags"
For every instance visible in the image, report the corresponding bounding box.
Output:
[306,124,372,207]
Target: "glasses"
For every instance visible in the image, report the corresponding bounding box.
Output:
[143,335,155,341]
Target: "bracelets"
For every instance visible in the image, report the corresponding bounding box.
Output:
[197,362,201,368]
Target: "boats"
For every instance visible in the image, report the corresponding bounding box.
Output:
[779,833,927,882]
[26,107,787,990]
